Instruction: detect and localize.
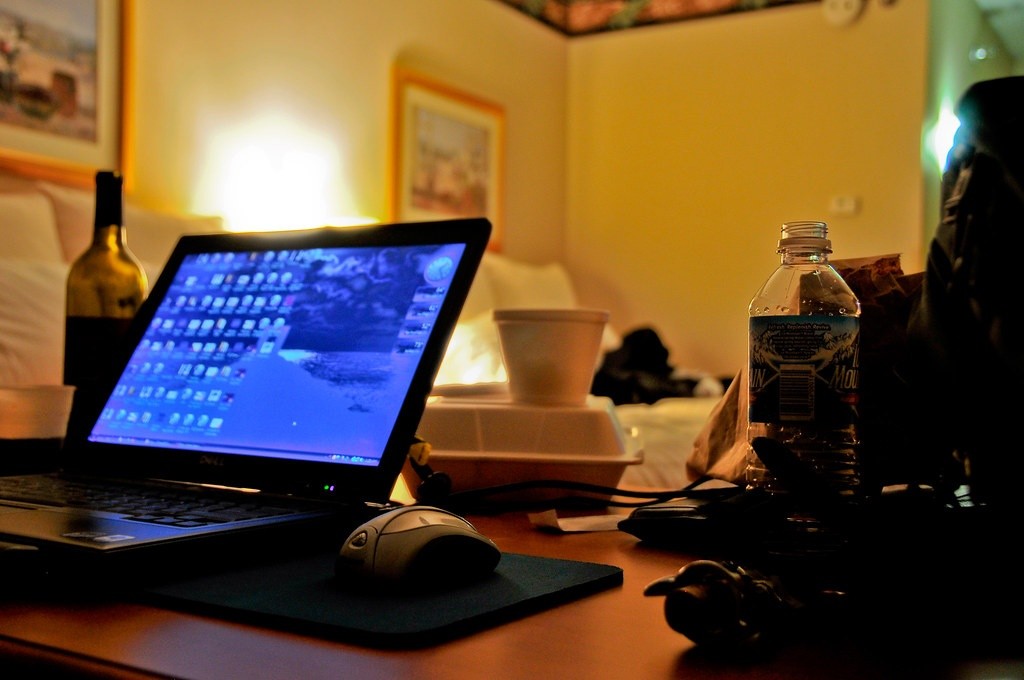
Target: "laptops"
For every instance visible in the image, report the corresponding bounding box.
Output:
[0,217,493,570]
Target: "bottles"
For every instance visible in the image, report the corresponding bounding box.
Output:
[64,170,148,455]
[745,220,858,559]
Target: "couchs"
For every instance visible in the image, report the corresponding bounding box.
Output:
[0,179,736,504]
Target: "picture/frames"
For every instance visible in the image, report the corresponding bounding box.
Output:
[0,0,136,194]
[388,64,509,252]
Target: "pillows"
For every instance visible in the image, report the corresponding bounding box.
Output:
[0,182,193,264]
[482,252,576,310]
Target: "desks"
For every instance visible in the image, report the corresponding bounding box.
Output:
[0,508,726,680]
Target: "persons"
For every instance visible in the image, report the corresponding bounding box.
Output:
[0,69,97,144]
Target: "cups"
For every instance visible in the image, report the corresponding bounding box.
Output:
[0,385,76,478]
[491,309,611,407]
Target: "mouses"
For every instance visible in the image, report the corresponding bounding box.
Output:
[337,506,503,580]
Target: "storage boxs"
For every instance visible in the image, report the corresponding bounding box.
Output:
[402,396,643,509]
